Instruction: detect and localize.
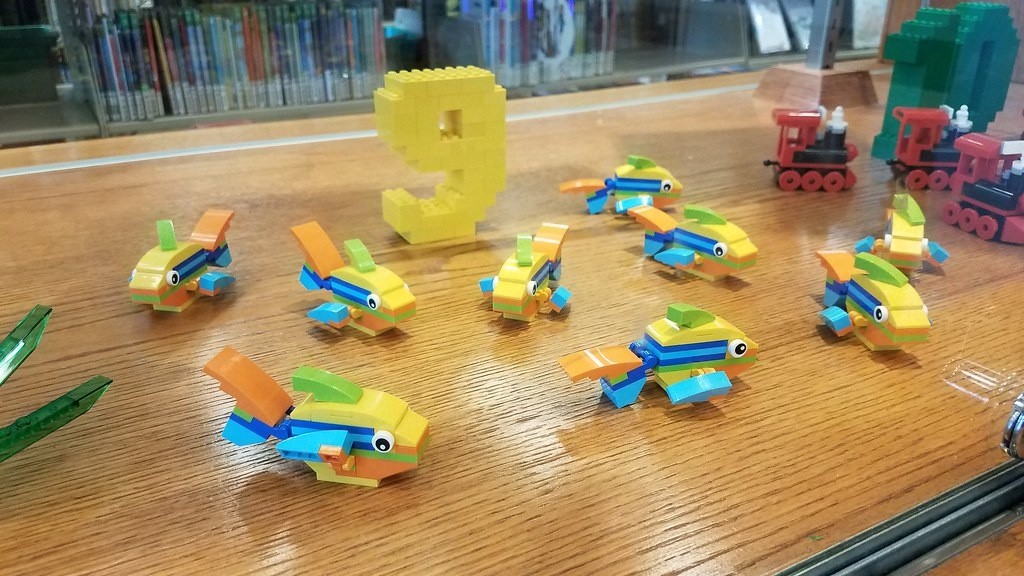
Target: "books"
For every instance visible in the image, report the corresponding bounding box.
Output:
[69,0,617,123]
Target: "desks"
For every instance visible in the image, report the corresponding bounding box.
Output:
[0,58,1024,576]
